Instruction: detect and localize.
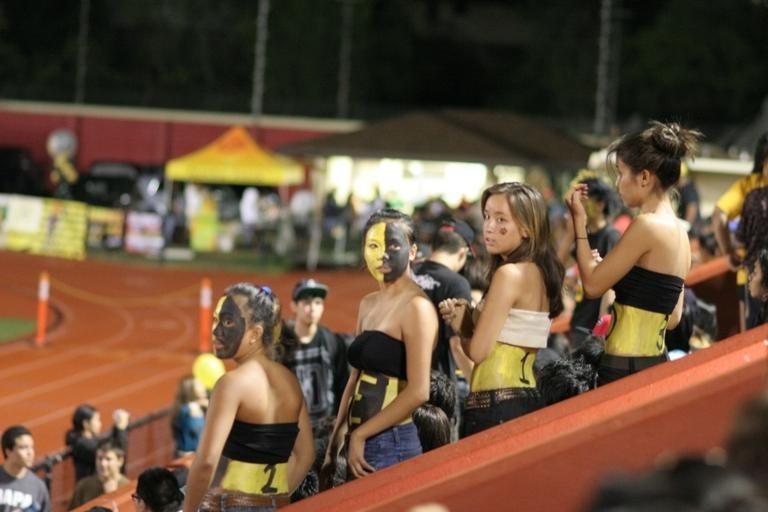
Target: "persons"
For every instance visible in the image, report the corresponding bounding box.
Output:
[435,119,767,438]
[588,408,768,511]
[170,204,475,510]
[289,190,475,271]
[1,404,185,511]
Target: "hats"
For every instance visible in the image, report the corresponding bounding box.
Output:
[293,279,327,300]
[437,217,478,261]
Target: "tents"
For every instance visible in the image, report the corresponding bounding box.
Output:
[165,126,303,243]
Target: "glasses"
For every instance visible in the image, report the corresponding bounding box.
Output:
[132,491,142,502]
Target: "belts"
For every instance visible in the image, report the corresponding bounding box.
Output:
[199,492,291,510]
[600,347,668,371]
[466,388,541,410]
